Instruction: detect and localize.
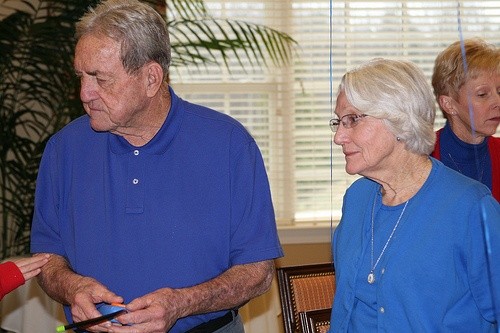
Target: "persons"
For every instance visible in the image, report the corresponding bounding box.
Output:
[0,255,51,302]
[325,59,500,333]
[30,0,285,333]
[429,38,500,204]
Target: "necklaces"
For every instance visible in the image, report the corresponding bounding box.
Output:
[368,192,408,285]
